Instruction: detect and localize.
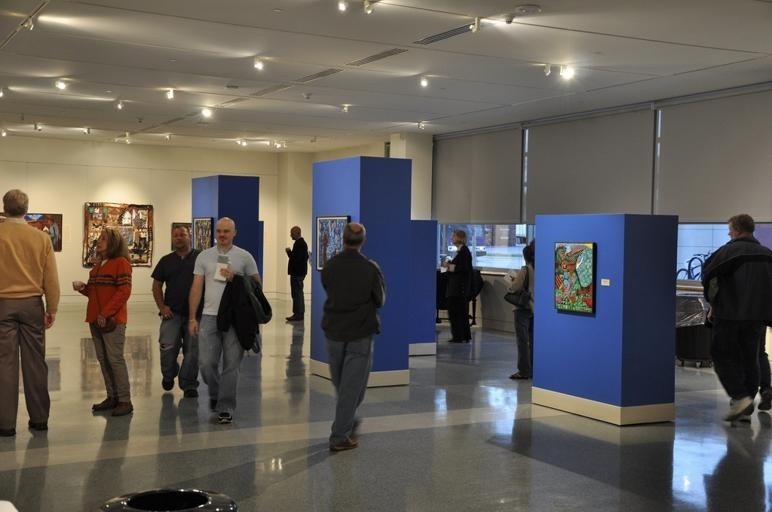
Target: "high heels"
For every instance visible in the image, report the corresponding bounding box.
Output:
[448,332,471,343]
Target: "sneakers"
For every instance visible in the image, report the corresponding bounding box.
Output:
[330,436,359,450]
[286,313,304,320]
[0,429,16,436]
[162,378,174,390]
[211,399,232,423]
[510,372,533,379]
[758,387,772,410]
[184,390,198,398]
[29,420,47,430]
[93,398,118,410]
[112,402,133,416]
[724,387,755,423]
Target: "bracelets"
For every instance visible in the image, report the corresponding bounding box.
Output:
[189,319,196,323]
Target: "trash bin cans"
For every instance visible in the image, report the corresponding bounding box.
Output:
[100,490,238,512]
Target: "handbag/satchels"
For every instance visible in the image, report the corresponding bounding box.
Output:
[504,289,530,307]
[95,317,116,332]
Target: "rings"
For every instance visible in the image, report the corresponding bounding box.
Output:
[165,314,167,317]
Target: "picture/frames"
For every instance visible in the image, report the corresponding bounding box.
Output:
[171,222,192,251]
[81,201,154,269]
[191,217,213,252]
[315,215,352,270]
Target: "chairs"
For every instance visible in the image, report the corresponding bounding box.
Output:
[676,294,718,367]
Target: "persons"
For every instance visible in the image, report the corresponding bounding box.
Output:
[150,225,205,398]
[700,214,772,428]
[321,222,386,452]
[188,216,261,424]
[72,227,134,416]
[442,230,472,344]
[0,188,60,436]
[507,238,535,380]
[46,217,60,251]
[285,226,309,321]
